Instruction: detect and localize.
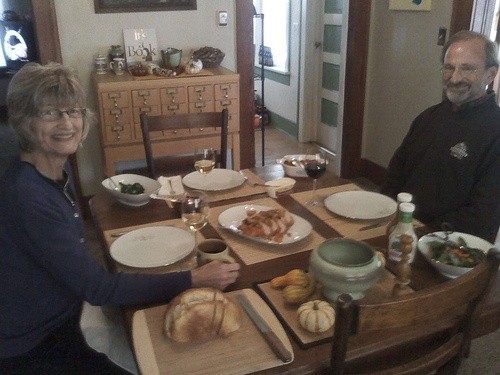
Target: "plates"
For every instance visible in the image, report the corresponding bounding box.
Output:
[217,204,314,246]
[323,190,398,219]
[110,227,195,269]
[183,169,245,192]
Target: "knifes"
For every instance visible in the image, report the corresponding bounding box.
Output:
[235,294,292,362]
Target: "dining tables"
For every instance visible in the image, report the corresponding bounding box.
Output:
[88,163,500,375]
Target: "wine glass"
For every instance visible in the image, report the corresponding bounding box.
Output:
[305,151,327,205]
[193,147,215,198]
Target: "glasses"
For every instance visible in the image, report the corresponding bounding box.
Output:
[29,108,87,121]
[439,66,485,75]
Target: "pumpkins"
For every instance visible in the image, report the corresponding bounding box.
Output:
[184,57,203,74]
[132,64,146,76]
[270,269,336,333]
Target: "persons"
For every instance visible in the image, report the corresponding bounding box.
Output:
[377,30,500,246]
[0,61,239,375]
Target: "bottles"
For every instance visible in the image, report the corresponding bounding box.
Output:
[109,45,124,62]
[384,193,413,268]
[385,202,417,289]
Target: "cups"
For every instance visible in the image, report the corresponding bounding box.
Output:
[112,58,127,75]
[180,191,209,229]
[93,56,108,75]
[195,239,235,267]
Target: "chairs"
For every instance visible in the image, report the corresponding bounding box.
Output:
[325,248,500,375]
[140,108,228,179]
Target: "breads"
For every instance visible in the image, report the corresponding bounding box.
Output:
[162,287,243,343]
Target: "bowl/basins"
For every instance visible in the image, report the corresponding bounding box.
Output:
[162,49,181,68]
[130,63,149,75]
[276,154,328,176]
[193,52,224,68]
[101,174,162,207]
[265,177,296,198]
[416,231,493,280]
[308,236,379,302]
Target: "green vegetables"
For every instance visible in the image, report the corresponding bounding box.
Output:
[429,236,485,268]
[119,182,145,195]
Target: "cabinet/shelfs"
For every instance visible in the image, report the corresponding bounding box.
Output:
[91,64,239,172]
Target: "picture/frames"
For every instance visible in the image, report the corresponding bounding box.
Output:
[94,0,197,14]
